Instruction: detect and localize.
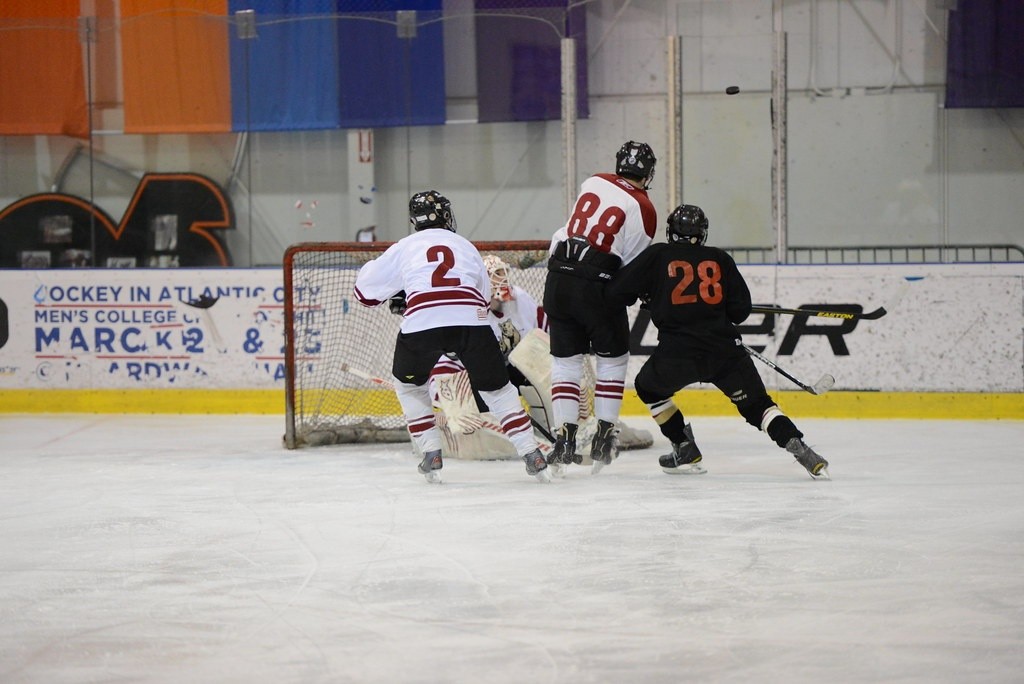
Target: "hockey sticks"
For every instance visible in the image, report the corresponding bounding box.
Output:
[752,284,897,320]
[742,343,836,396]
[528,415,561,446]
[343,368,563,465]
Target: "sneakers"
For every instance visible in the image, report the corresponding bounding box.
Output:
[785,438,829,480]
[418,449,443,484]
[589,419,621,475]
[522,448,551,482]
[547,422,579,477]
[659,422,707,474]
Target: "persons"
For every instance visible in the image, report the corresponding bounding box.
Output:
[623,204,830,479]
[428,226,653,460]
[549,140,659,472]
[353,191,551,483]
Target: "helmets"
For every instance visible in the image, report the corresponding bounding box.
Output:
[665,204,708,246]
[615,140,657,190]
[409,190,457,233]
[481,255,513,301]
